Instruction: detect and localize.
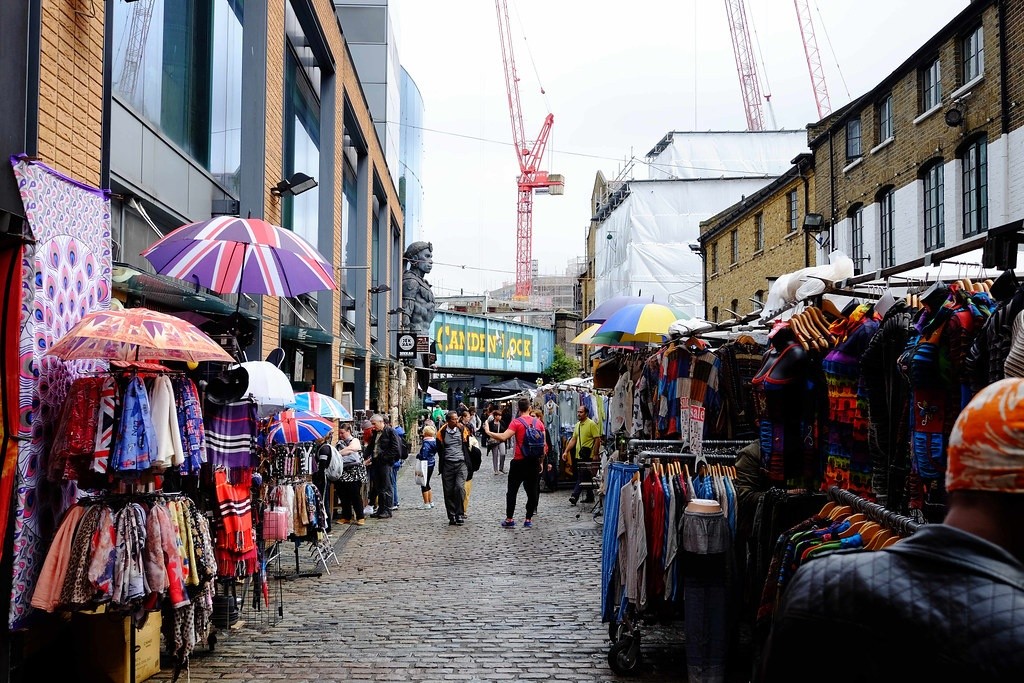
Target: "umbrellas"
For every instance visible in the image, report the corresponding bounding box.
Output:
[569,289,692,381]
[260,385,353,445]
[138,209,340,336]
[43,300,236,375]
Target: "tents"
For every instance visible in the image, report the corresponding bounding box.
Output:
[427,387,447,416]
[468,376,537,398]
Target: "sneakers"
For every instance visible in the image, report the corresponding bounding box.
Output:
[501,521,515,526]
[378,508,392,517]
[418,503,431,510]
[336,519,350,524]
[370,508,383,518]
[354,519,365,525]
[524,521,533,527]
[420,502,434,507]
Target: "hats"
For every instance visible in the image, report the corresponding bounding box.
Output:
[946,377,1024,492]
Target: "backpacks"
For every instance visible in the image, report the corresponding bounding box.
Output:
[386,426,408,460]
[515,418,544,457]
[318,444,343,481]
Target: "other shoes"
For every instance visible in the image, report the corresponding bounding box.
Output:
[499,469,507,474]
[569,497,577,504]
[449,516,456,525]
[495,471,498,475]
[580,496,594,503]
[456,515,465,524]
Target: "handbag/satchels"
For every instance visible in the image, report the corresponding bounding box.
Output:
[414,459,428,487]
[579,447,591,458]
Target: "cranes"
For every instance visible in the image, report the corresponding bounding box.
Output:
[495,1,565,302]
[723,0,770,132]
[795,0,853,118]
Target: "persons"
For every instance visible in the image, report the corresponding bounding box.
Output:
[562,405,600,506]
[745,270,1024,526]
[762,378,1024,683]
[329,399,554,529]
[402,241,436,336]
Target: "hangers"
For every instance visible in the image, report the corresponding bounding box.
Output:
[76,370,187,378]
[543,384,607,396]
[791,486,923,562]
[595,326,771,374]
[621,440,754,503]
[77,491,187,505]
[546,396,559,408]
[785,262,995,351]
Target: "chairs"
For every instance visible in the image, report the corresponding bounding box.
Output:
[576,461,602,512]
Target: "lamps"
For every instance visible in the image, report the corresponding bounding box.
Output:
[802,213,825,233]
[368,284,391,293]
[270,173,318,197]
[401,323,416,328]
[689,244,704,262]
[388,307,405,315]
